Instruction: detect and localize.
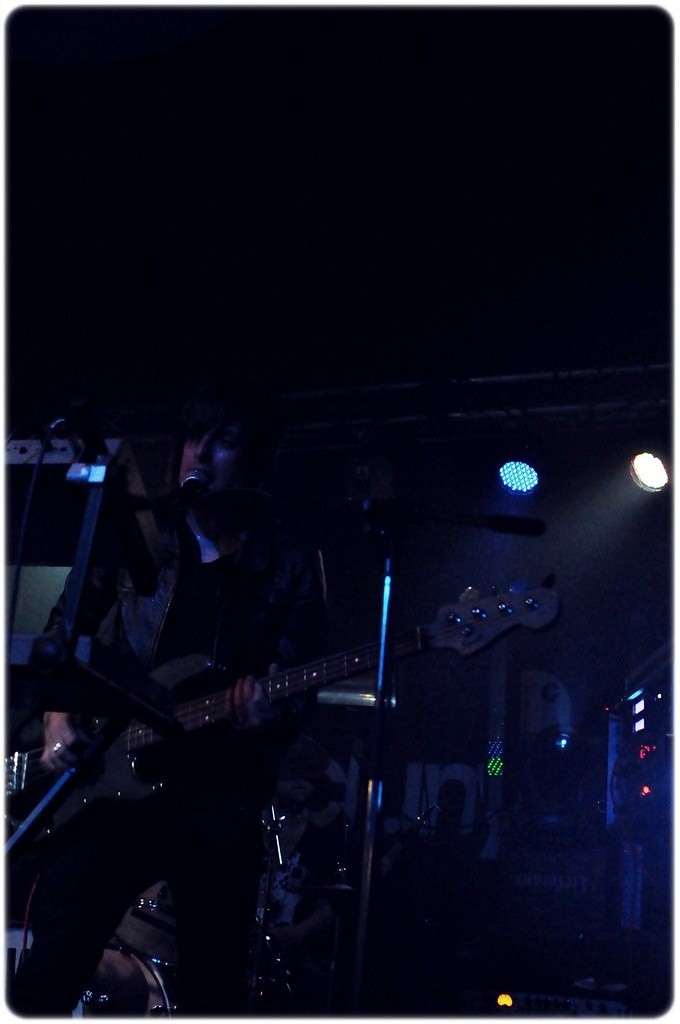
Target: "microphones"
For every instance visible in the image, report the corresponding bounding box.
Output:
[170,469,209,512]
[72,660,185,742]
[488,508,545,538]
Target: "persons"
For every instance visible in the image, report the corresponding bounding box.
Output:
[8,373,329,1015]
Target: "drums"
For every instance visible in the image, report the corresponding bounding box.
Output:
[8,919,175,1016]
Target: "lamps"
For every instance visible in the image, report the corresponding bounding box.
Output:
[496,432,551,498]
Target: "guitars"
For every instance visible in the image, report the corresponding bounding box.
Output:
[6,578,563,860]
